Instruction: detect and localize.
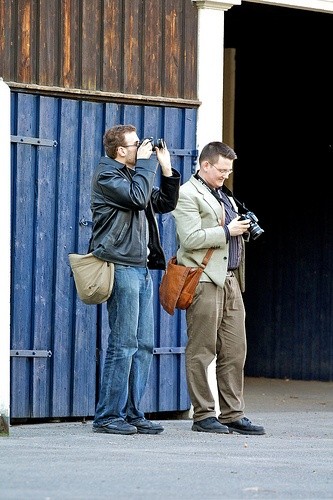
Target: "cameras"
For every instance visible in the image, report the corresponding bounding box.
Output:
[138,137,164,151]
[240,212,265,241]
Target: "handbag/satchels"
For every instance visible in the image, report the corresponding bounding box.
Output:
[159,257,203,315]
[68,251,114,305]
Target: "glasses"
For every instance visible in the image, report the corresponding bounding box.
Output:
[121,141,140,147]
[208,160,232,175]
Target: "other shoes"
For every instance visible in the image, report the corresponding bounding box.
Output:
[93,421,137,434]
[218,417,265,434]
[124,417,164,434]
[192,417,229,433]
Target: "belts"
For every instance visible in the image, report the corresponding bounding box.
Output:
[227,270,235,276]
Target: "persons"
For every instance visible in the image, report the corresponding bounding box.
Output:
[173,141,265,436]
[87,125,182,433]
